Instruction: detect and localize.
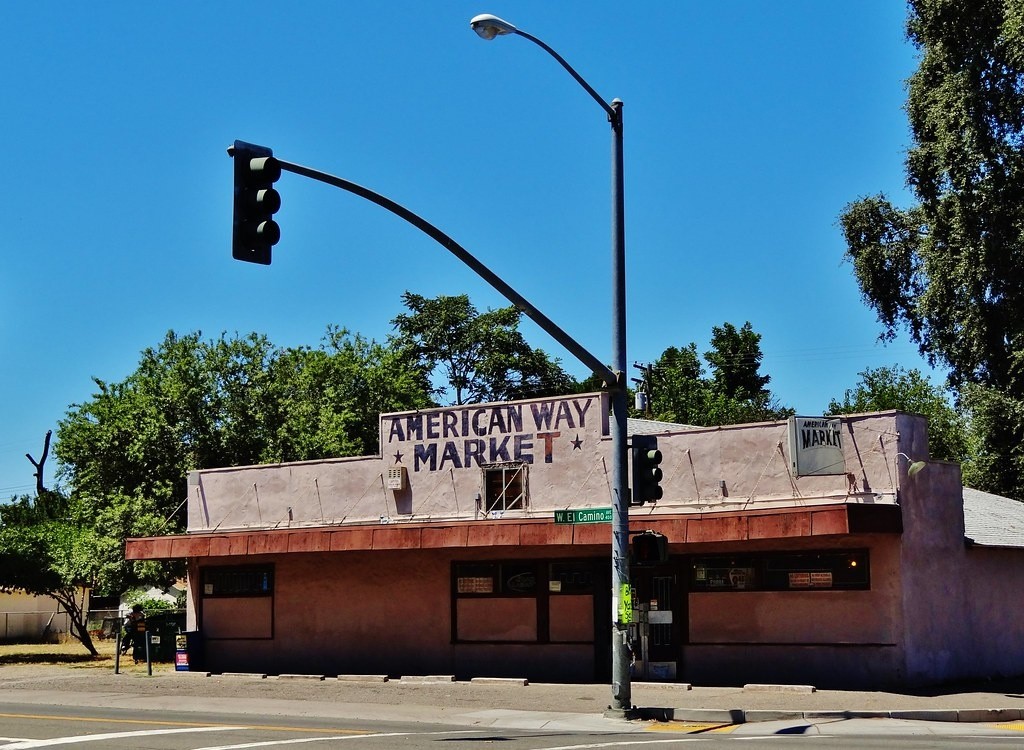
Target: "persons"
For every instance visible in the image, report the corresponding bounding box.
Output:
[122,604,146,664]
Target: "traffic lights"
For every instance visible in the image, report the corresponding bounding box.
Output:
[232,139,281,265]
[632,434,663,501]
[633,532,668,564]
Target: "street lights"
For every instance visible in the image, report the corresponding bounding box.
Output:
[469,13,636,718]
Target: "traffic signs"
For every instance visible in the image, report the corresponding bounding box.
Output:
[554,508,613,524]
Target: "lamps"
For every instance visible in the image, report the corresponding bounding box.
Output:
[894,453,926,497]
